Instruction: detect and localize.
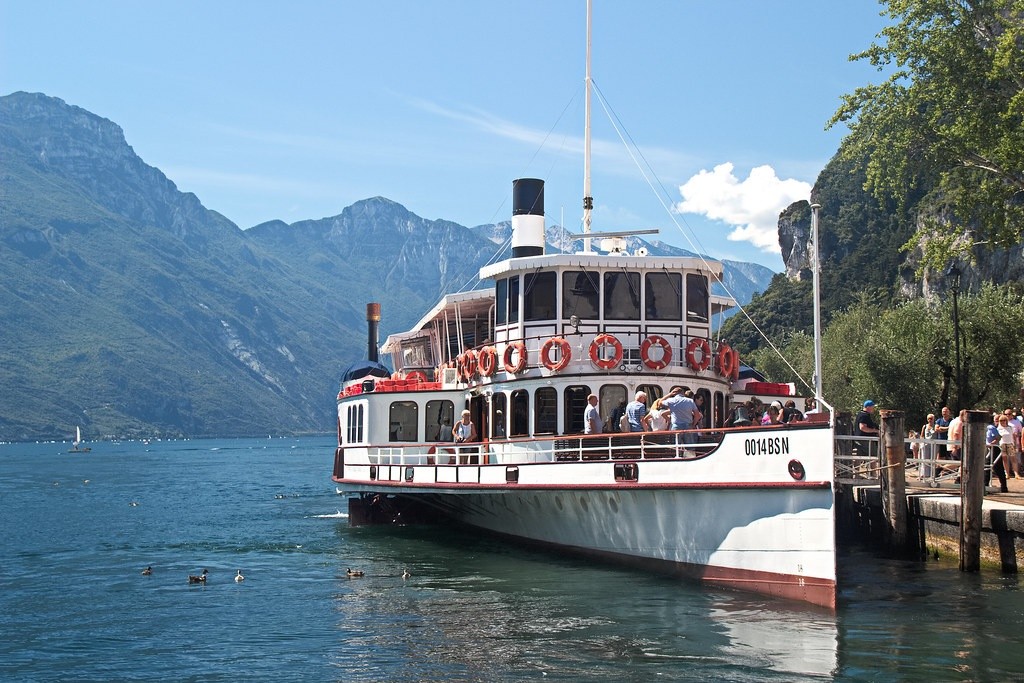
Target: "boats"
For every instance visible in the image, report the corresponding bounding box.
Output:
[331,0,837,612]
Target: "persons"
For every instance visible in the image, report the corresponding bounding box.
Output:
[947,409,964,484]
[452,410,477,464]
[610,387,704,457]
[854,400,879,480]
[909,429,920,459]
[935,407,953,460]
[436,417,452,441]
[921,414,940,478]
[986,408,1024,492]
[584,394,603,435]
[745,396,803,426]
[497,410,502,436]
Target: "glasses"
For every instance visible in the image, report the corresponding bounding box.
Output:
[1000,420,1006,422]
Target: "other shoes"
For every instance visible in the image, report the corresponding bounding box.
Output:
[1000,487,1008,493]
[1005,475,1010,479]
[868,474,878,480]
[1015,475,1024,480]
[955,477,961,484]
[859,472,868,479]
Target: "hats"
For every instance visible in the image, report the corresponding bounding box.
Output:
[864,400,876,408]
[770,401,782,410]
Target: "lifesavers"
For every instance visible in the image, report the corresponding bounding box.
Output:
[449,361,455,368]
[458,355,464,382]
[685,338,711,372]
[434,368,440,382]
[503,342,528,374]
[589,334,623,370]
[733,350,739,381]
[441,363,448,382]
[427,441,456,464]
[478,346,495,377]
[541,338,572,372]
[462,350,476,380]
[715,343,733,377]
[405,371,428,382]
[640,336,672,369]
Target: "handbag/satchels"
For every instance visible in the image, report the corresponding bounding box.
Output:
[619,413,630,433]
[761,411,772,426]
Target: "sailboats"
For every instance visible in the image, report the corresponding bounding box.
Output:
[68,425,92,453]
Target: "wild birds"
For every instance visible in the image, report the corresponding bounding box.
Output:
[402,569,411,580]
[188,569,209,583]
[346,568,365,577]
[128,502,142,507]
[234,570,244,583]
[141,566,152,575]
